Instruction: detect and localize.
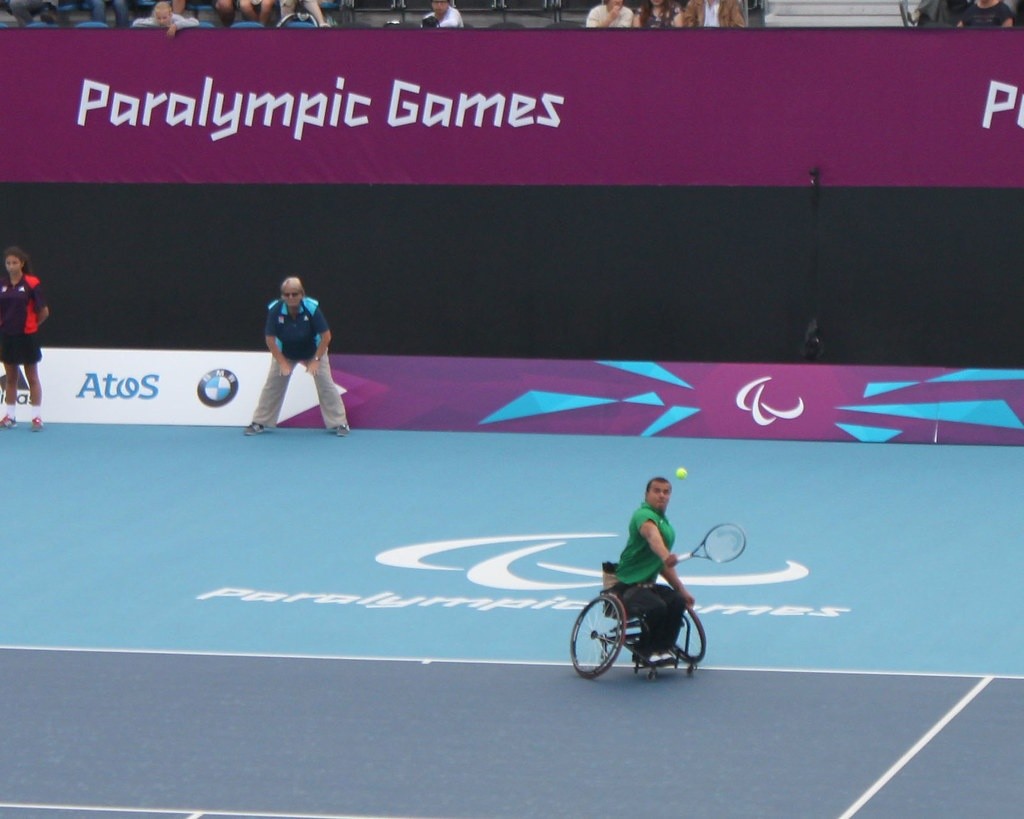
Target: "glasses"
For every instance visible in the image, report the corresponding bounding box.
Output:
[283,290,302,297]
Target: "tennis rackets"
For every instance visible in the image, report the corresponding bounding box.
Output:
[677,522,747,564]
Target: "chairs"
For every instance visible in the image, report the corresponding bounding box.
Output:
[0,0,758,30]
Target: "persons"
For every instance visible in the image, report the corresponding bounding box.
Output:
[616,477,695,661]
[10,0,128,25]
[132,2,199,39]
[172,0,235,23]
[245,278,350,437]
[421,0,463,28]
[239,0,275,22]
[633,0,683,27]
[908,0,1016,28]
[586,0,634,27]
[279,0,330,27]
[685,0,745,28]
[0,246,49,430]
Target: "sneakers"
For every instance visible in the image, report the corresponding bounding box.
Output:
[32,417,43,432]
[0,415,17,429]
[336,424,351,436]
[245,423,264,436]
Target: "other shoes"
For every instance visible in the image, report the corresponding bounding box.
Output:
[649,653,664,666]
[661,652,677,664]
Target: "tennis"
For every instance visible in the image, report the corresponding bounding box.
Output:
[675,468,687,480]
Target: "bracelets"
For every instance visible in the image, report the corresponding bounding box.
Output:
[314,357,320,361]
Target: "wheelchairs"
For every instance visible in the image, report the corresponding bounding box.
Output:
[570,560,708,679]
